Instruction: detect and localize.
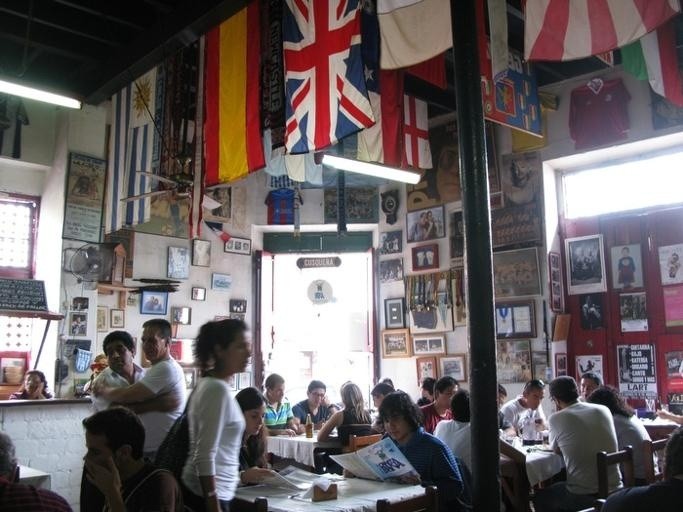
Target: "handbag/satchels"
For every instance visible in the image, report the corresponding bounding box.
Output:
[153,412,190,482]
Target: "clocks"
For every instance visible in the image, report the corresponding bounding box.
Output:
[380,189,400,224]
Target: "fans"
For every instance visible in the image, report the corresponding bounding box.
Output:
[67,239,115,285]
[119,75,239,210]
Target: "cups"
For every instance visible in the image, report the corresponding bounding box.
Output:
[661,404,669,411]
[516,417,537,441]
[644,399,656,413]
[542,435,549,449]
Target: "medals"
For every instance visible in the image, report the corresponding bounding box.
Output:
[406,300,461,313]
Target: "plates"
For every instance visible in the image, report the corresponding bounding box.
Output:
[536,445,553,452]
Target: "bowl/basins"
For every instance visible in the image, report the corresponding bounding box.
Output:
[3,366,23,384]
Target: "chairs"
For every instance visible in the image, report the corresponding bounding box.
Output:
[229,418,682,512]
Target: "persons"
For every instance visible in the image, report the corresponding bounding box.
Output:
[407,210,439,242]
[503,156,538,208]
[582,294,602,329]
[616,246,636,288]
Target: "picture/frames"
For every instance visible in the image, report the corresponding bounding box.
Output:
[416,356,439,388]
[381,329,413,359]
[70,310,88,338]
[375,204,470,334]
[485,151,542,389]
[109,309,124,329]
[98,304,108,333]
[61,151,108,243]
[609,242,645,290]
[414,333,447,357]
[162,239,253,391]
[437,354,468,383]
[405,119,502,213]
[142,287,167,315]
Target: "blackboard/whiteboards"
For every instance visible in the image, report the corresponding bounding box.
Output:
[0,278,47,311]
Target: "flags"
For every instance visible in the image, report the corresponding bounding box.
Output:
[104,63,166,235]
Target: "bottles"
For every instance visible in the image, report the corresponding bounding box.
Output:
[304,414,313,438]
